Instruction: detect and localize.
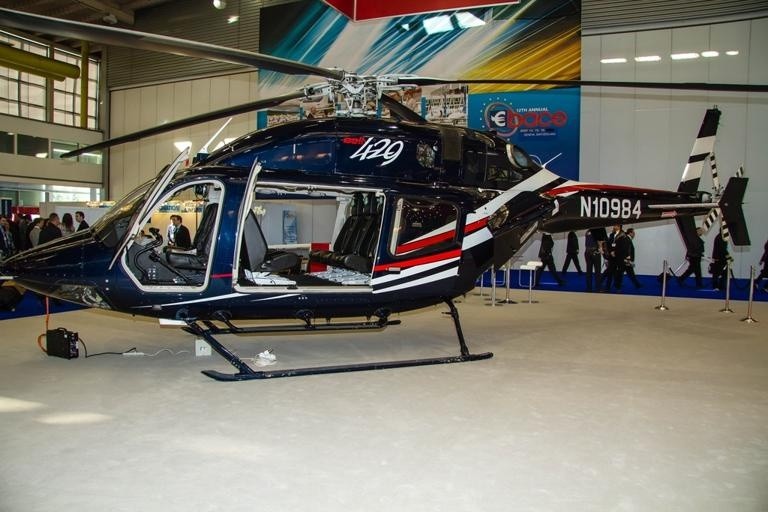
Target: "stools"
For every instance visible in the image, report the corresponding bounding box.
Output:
[473,258,543,310]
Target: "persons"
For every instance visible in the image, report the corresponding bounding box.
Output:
[712,227,728,290]
[678,227,707,289]
[167,215,191,251]
[134,230,150,247]
[532,233,566,287]
[307,107,317,120]
[585,224,643,292]
[0,211,89,261]
[754,240,768,293]
[562,230,585,276]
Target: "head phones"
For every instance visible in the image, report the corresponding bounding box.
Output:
[194,184,209,197]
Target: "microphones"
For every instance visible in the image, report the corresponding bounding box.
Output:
[193,194,209,201]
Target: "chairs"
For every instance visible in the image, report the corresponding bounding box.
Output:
[163,201,380,278]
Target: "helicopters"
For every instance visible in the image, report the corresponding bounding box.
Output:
[1,7,767,382]
